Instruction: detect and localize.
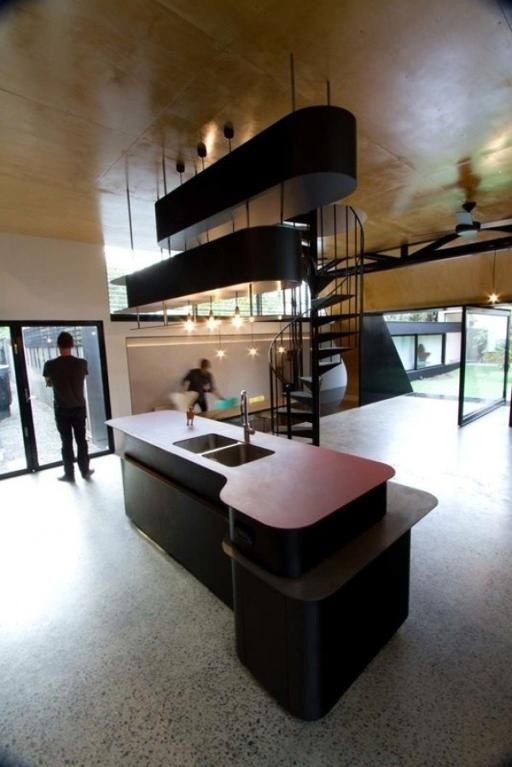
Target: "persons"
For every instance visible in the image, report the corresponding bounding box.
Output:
[43,331,95,483]
[180,357,225,414]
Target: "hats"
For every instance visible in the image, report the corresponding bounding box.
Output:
[57,332,74,347]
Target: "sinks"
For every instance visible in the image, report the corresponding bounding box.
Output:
[174,432,238,453]
[203,441,275,467]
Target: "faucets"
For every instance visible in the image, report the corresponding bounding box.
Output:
[239,390,255,443]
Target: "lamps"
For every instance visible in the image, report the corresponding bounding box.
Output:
[177,124,243,330]
[457,201,480,236]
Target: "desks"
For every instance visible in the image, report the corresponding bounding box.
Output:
[196,396,298,421]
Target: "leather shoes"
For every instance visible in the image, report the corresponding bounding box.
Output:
[57,474,75,482]
[81,468,94,478]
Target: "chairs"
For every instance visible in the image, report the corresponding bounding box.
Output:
[216,397,241,424]
[249,396,276,432]
